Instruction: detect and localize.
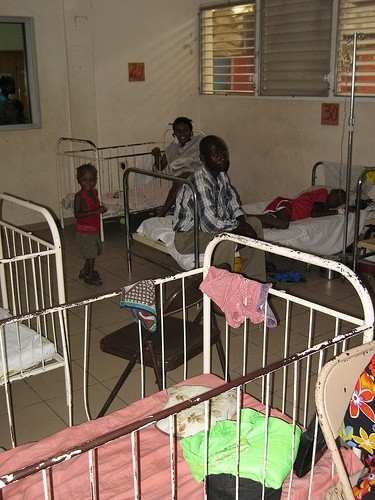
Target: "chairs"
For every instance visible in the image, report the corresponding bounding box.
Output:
[314,341,375,500]
[94,262,232,419]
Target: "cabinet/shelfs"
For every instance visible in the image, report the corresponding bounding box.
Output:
[353,167,375,273]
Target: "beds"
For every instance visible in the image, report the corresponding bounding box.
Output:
[122,161,375,276]
[56,129,207,242]
[0,234,375,500]
[0,193,73,449]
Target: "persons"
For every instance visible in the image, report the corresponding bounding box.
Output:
[152,117,205,218]
[246,188,346,229]
[74,162,107,285]
[172,135,266,317]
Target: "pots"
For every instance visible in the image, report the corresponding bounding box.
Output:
[319,256,342,280]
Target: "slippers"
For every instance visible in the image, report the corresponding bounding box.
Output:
[266,268,308,286]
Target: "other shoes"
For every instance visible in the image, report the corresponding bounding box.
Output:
[78,270,104,286]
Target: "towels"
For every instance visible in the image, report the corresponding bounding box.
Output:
[119,279,157,333]
